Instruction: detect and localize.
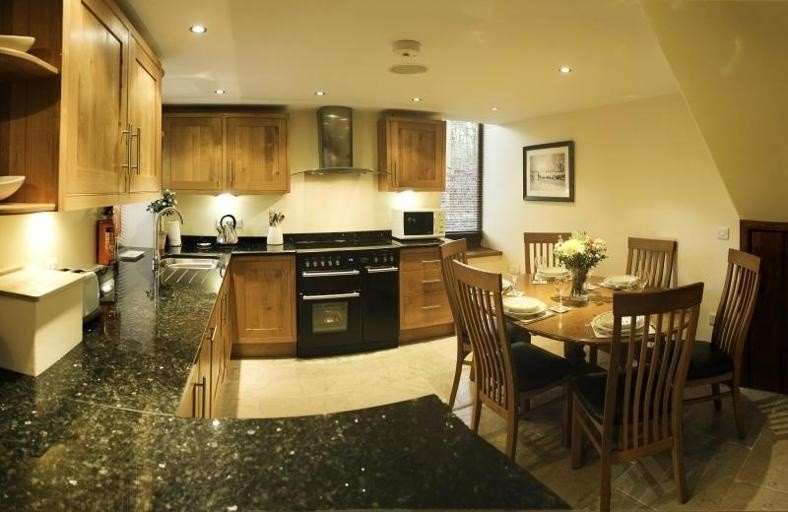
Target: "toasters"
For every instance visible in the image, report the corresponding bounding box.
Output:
[57,267,101,325]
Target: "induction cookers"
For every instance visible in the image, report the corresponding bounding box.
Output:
[294,239,397,272]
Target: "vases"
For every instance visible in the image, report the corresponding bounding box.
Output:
[158,232,168,251]
[567,268,589,303]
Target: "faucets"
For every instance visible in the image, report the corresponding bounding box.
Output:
[154,209,184,254]
[153,271,182,318]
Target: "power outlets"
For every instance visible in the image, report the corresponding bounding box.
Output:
[708,311,716,324]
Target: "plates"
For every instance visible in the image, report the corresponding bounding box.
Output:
[603,274,638,291]
[497,294,547,317]
[539,267,569,280]
[592,310,646,333]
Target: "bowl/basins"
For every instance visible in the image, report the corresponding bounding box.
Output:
[1,174,27,203]
[1,33,36,53]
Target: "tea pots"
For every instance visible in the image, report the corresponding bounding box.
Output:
[215,214,239,246]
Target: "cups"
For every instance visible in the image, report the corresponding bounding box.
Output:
[533,255,547,267]
[266,226,284,246]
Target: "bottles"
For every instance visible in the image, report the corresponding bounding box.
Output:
[96,213,115,266]
[169,221,181,247]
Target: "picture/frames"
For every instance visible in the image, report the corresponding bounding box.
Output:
[523,141,575,203]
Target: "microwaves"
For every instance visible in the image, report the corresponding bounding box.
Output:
[391,207,445,240]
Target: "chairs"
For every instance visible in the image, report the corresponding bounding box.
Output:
[656,248,763,439]
[626,236,676,290]
[523,232,572,274]
[439,238,531,410]
[451,259,571,461]
[567,281,703,512]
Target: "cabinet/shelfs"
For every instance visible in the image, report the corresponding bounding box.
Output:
[1,0,164,215]
[232,254,298,358]
[161,112,225,197]
[399,248,456,342]
[218,263,231,387]
[225,112,291,196]
[198,303,218,417]
[376,116,446,192]
[176,354,206,419]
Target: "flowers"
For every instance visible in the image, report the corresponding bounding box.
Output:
[553,232,607,297]
[146,190,178,230]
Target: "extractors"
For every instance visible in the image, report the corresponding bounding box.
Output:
[287,105,393,177]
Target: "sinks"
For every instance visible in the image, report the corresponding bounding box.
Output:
[159,258,220,270]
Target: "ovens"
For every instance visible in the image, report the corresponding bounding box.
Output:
[296,267,400,357]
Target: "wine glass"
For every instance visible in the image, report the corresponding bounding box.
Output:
[505,262,522,298]
[552,273,573,312]
